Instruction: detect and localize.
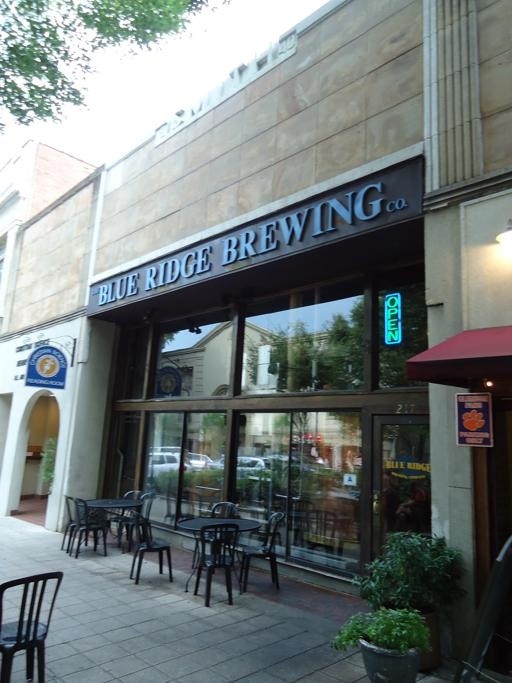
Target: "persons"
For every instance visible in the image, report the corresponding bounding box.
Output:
[382,474,431,534]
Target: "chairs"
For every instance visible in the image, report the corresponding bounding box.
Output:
[0,570,65,682]
[60,485,360,606]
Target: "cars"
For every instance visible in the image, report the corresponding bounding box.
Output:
[146,445,390,538]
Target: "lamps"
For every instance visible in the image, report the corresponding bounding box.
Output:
[490,215,511,244]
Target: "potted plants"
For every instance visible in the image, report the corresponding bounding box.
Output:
[351,529,466,673]
[329,604,437,682]
